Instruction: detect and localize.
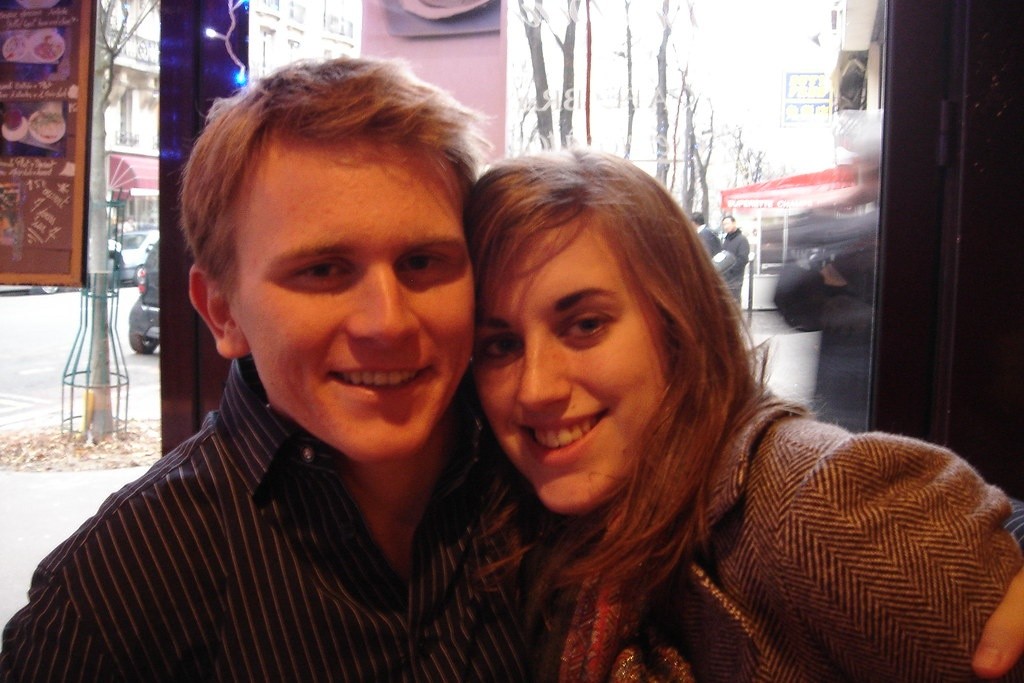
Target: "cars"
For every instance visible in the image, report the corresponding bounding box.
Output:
[129,239,159,356]
[114,231,160,284]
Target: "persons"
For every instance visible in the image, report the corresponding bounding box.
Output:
[690,212,719,259]
[773,131,883,435]
[719,216,750,303]
[460,150,1023,682]
[0,54,537,683]
